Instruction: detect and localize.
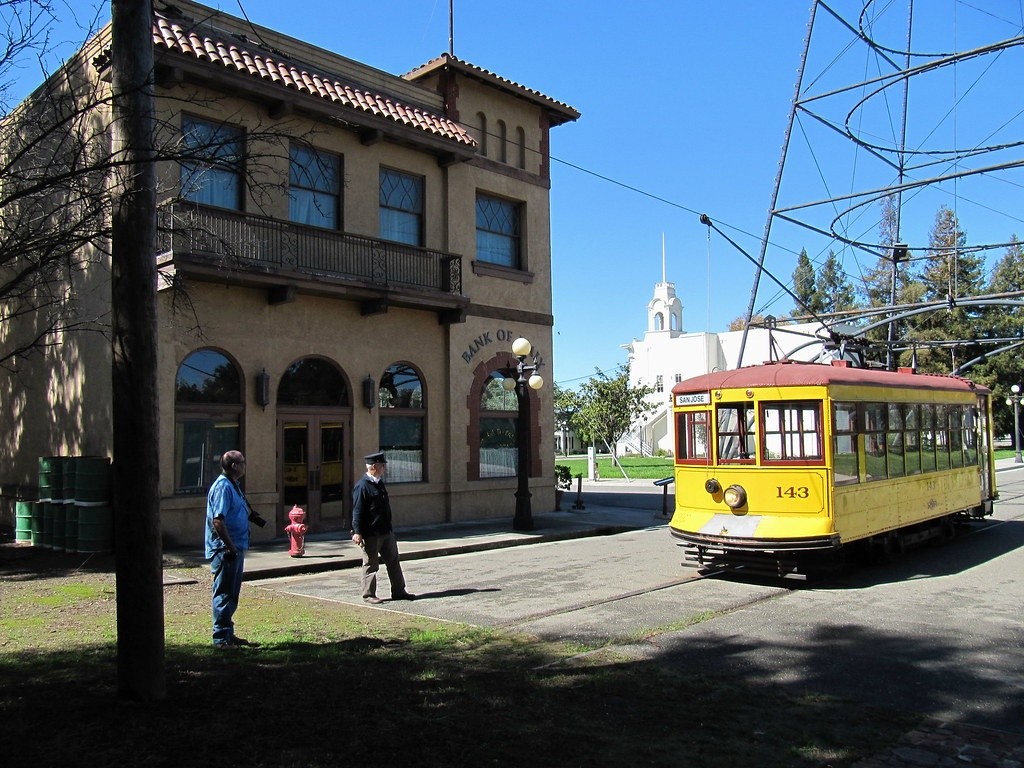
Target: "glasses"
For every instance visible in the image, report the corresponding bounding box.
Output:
[230,458,246,466]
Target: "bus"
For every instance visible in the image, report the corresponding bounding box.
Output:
[670,214,1000,577]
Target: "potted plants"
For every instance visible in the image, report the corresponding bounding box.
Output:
[555,465,573,511]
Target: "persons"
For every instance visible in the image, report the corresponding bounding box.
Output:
[204,451,251,645]
[352,452,416,604]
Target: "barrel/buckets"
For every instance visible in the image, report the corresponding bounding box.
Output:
[37,455,111,502]
[15,501,113,553]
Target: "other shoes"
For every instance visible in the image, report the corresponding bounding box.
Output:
[216,640,244,656]
[392,590,415,600]
[229,635,249,646]
[366,597,383,604]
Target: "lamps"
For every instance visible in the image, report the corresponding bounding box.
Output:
[363,374,374,413]
[257,368,269,411]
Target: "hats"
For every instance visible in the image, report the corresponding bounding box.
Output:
[364,452,388,464]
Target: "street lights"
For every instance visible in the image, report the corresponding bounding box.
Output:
[557,420,569,455]
[501,337,544,531]
[1006,384,1024,463]
